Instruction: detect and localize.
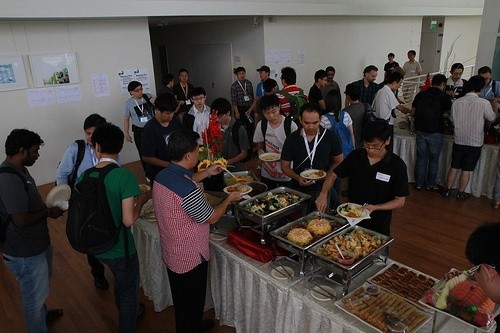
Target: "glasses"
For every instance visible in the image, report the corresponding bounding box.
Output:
[360,139,386,151]
[465,264,495,274]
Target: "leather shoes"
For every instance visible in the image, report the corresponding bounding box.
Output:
[41,308,64,322]
[194,319,215,332]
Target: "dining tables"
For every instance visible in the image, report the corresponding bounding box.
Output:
[391,103,500,150]
[129,196,499,333]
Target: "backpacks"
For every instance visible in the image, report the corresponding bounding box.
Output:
[0,197,9,251]
[322,110,354,156]
[66,164,120,256]
[279,90,307,121]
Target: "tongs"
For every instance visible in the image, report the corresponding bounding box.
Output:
[269,260,294,281]
[303,281,338,302]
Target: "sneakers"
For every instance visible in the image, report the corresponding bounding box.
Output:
[123,302,144,328]
[414,185,424,191]
[439,188,450,198]
[427,186,443,192]
[456,191,470,200]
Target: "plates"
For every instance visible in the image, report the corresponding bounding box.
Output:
[210,230,228,241]
[333,281,434,333]
[336,202,370,219]
[367,261,443,313]
[138,184,151,193]
[271,266,295,280]
[45,185,72,209]
[259,153,282,162]
[418,286,496,333]
[223,184,253,195]
[311,285,336,302]
[300,169,327,180]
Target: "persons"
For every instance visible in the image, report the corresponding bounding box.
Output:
[462,222,500,333]
[443,63,467,105]
[75,122,148,333]
[477,67,500,104]
[440,74,500,200]
[152,128,244,333]
[121,49,424,182]
[313,118,411,256]
[55,114,122,290]
[0,128,68,333]
[281,102,345,215]
[409,74,453,192]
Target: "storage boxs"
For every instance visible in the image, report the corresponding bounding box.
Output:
[307,224,394,279]
[269,210,352,256]
[237,186,311,225]
